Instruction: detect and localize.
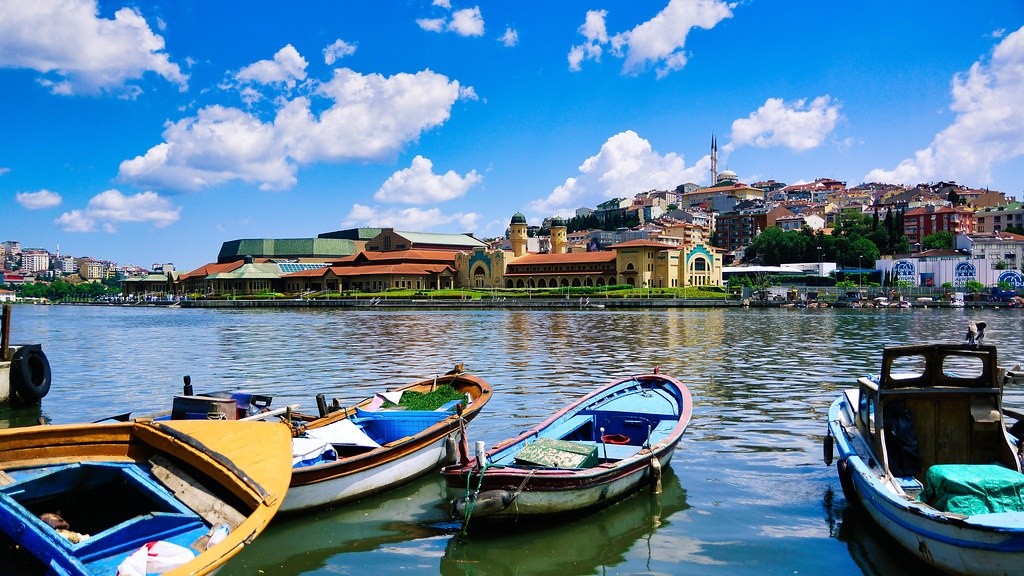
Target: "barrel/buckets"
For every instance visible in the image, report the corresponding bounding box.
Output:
[601,435,630,445]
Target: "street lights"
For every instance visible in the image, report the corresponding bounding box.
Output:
[860,256,862,288]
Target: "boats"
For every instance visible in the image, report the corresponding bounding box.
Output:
[847,298,912,308]
[779,301,829,309]
[827,323,1024,576]
[91,363,493,518]
[0,417,293,576]
[949,300,965,308]
[440,366,692,525]
